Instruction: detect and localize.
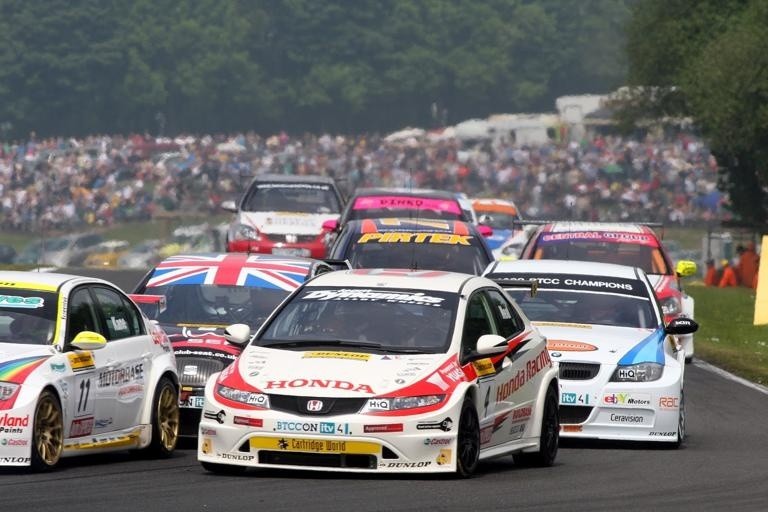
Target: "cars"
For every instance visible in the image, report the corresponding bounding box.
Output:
[0,268,181,472]
[0,220,223,267]
[198,269,560,475]
[513,219,699,362]
[321,173,525,271]
[130,252,352,436]
[480,260,699,449]
[220,173,346,260]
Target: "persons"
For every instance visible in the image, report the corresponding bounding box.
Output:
[4,123,746,228]
[693,241,762,287]
